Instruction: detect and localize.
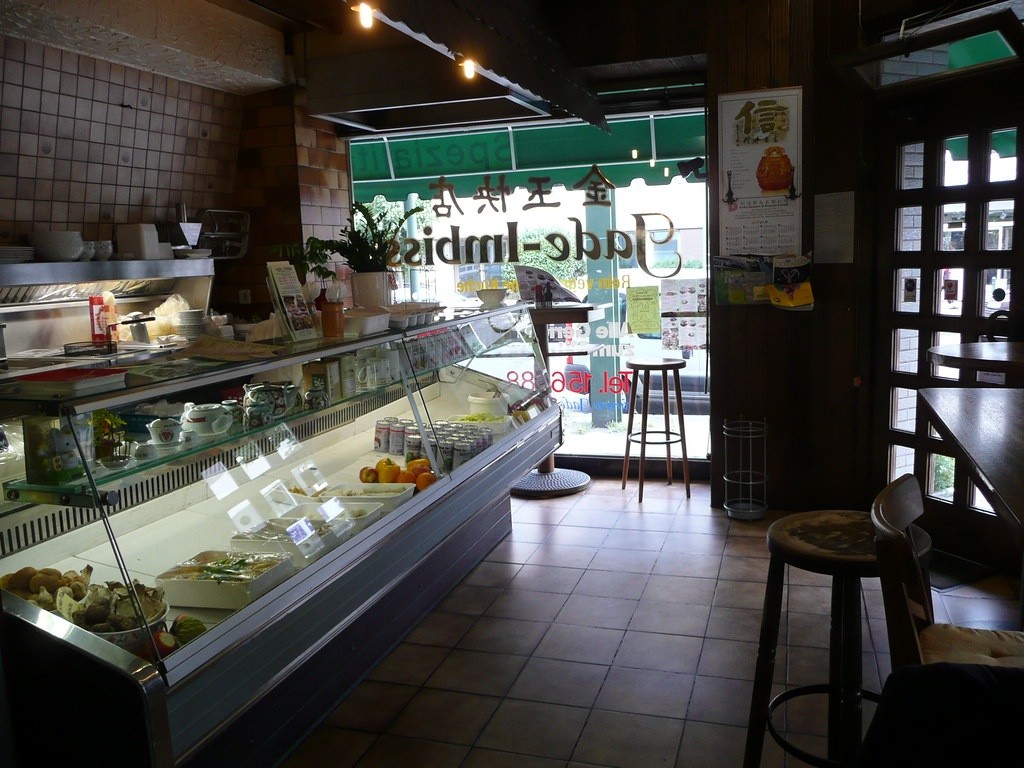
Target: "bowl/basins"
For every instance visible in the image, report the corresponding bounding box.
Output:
[82,240,95,261]
[95,240,113,261]
[93,598,170,650]
[174,249,212,259]
[38,230,83,262]
[476,289,508,312]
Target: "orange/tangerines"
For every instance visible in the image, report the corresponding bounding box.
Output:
[396,471,435,491]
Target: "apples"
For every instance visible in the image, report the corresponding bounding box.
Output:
[409,464,430,476]
[359,466,377,483]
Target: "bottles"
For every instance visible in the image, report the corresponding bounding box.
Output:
[90,296,106,348]
[126,311,151,344]
[101,292,120,344]
[321,302,345,340]
[401,326,466,368]
[535,282,553,309]
[510,389,549,413]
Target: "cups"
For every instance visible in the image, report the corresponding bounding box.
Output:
[134,445,158,460]
[145,415,184,444]
[358,357,390,389]
[221,400,240,425]
[305,388,329,410]
[180,431,199,449]
[243,403,275,433]
[377,349,399,382]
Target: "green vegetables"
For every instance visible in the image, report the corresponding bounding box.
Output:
[455,412,505,423]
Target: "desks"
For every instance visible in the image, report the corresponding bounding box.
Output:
[511,302,594,498]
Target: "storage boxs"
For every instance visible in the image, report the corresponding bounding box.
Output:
[319,483,416,514]
[22,412,96,486]
[280,502,384,532]
[230,517,354,568]
[273,481,333,505]
[155,550,293,608]
[302,344,381,405]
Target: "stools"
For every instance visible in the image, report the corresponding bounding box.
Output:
[622,358,690,503]
[743,509,935,768]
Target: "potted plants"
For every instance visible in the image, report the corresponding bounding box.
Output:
[268,200,424,307]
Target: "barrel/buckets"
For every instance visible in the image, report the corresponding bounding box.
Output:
[467,392,509,416]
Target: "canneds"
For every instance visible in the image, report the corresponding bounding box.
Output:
[373,416,494,472]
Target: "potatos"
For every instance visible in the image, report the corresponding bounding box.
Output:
[7,567,138,632]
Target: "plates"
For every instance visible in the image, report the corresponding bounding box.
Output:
[0,245,35,264]
[174,309,205,339]
[116,223,160,260]
[146,439,184,450]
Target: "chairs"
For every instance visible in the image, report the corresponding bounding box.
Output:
[871,474,1024,672]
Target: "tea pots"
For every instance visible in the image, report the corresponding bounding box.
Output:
[180,402,233,435]
[243,381,301,419]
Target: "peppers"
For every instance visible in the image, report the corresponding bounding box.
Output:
[375,457,400,483]
